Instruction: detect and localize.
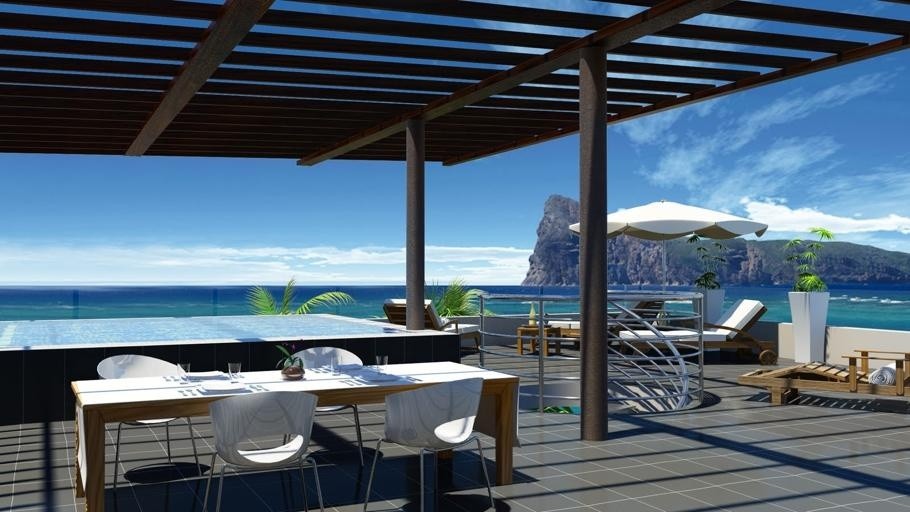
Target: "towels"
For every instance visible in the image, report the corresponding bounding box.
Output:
[869,361,897,383]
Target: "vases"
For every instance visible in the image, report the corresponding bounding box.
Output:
[281,364,305,380]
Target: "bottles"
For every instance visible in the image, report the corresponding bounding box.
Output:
[529,302,536,326]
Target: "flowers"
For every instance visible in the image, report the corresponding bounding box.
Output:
[273,337,308,369]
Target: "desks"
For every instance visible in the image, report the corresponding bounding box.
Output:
[70,360,521,511]
[516,324,562,358]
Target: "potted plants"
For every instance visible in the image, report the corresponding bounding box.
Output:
[687,231,728,352]
[784,227,836,363]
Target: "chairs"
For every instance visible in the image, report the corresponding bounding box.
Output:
[202,391,327,511]
[383,297,484,353]
[279,347,364,467]
[94,354,202,495]
[618,298,778,367]
[536,296,665,354]
[736,349,909,405]
[364,378,496,511]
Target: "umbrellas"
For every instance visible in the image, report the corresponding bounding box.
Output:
[568,200,768,314]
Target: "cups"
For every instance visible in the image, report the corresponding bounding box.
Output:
[330,354,342,375]
[376,354,389,377]
[176,362,190,385]
[228,362,242,384]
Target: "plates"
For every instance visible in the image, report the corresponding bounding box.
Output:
[323,363,402,381]
[183,367,245,391]
